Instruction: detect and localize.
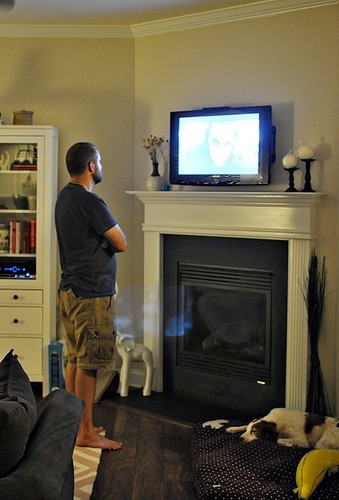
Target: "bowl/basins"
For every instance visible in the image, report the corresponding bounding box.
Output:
[13,194,28,210]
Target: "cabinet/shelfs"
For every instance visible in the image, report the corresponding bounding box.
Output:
[0,124,59,399]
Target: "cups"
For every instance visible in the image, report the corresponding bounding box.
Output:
[0,223,9,253]
[27,196,36,210]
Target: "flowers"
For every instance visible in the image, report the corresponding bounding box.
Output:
[143,134,169,162]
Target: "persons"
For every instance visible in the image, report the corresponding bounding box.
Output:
[53,141,127,451]
[10,149,36,170]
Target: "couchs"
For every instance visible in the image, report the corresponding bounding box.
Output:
[0,349,86,500]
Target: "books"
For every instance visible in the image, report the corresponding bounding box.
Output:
[0,220,36,255]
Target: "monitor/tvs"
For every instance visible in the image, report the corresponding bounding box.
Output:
[169,105,272,186]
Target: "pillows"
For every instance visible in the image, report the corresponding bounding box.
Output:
[0,349,37,478]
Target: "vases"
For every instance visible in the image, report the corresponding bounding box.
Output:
[146,163,164,191]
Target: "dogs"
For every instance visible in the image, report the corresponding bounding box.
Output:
[226,406,339,450]
